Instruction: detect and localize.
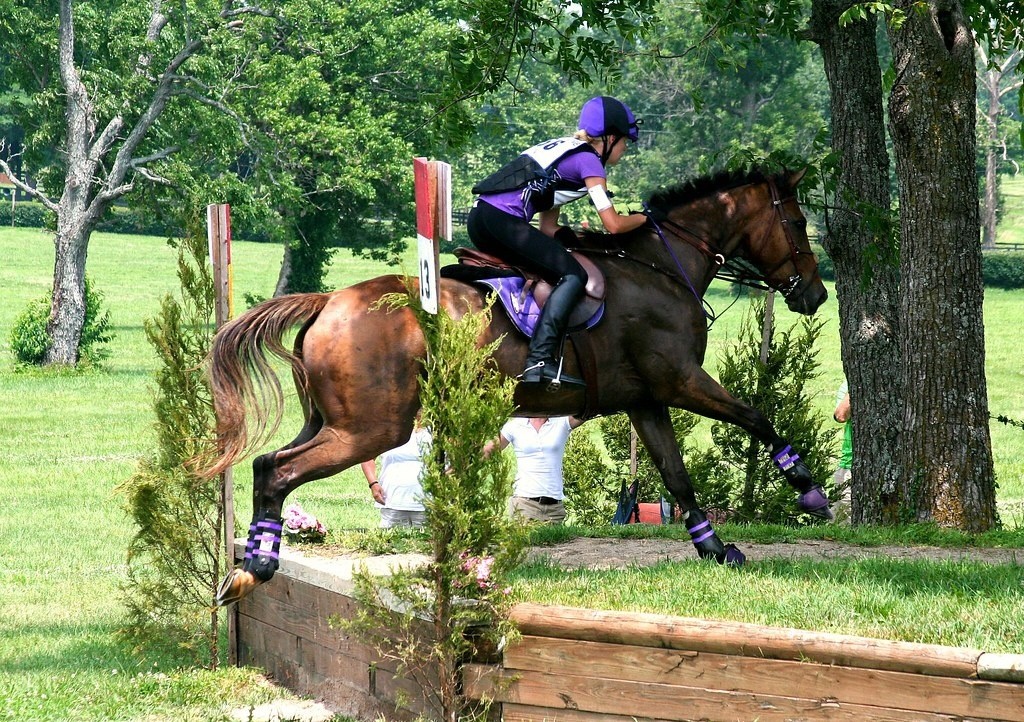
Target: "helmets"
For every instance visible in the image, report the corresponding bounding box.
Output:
[578,95,640,141]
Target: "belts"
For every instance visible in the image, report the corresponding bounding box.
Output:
[530,496,560,505]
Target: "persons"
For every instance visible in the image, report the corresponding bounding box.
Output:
[359,407,459,534]
[483,415,591,527]
[466,96,662,393]
[826,376,853,527]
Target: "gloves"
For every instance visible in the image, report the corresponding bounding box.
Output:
[554,226,579,247]
[643,207,664,222]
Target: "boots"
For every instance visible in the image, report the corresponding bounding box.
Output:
[517,275,587,392]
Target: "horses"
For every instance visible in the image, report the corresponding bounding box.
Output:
[178,158,835,618]
